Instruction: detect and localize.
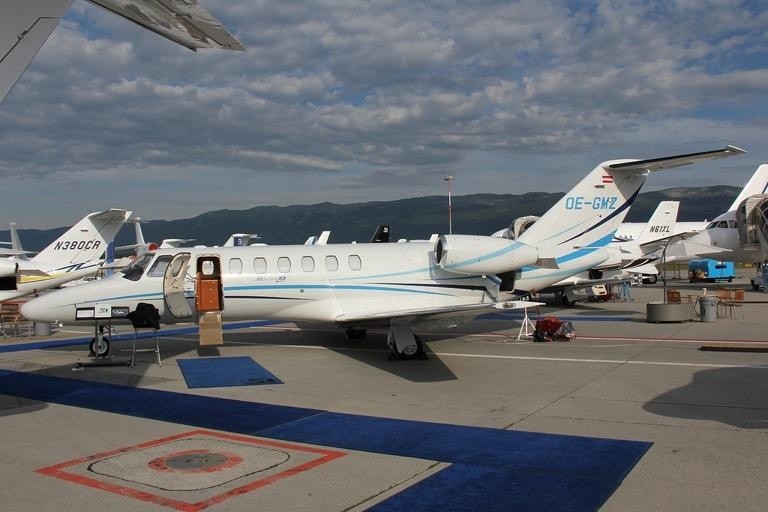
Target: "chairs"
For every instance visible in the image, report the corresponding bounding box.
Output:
[717,286,746,319]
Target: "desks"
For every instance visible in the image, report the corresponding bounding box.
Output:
[687,293,700,316]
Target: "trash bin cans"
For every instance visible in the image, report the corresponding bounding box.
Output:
[699,296,717,323]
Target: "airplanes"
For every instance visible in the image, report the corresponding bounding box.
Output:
[1,0,249,307]
